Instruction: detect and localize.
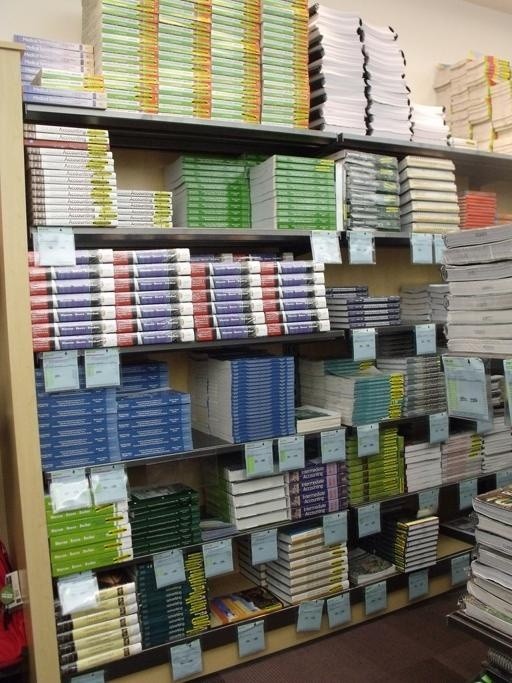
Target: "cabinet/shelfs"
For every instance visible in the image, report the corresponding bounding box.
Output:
[0,42,511,682]
[438,348,511,683]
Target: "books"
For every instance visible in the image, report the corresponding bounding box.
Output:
[440,223,512,355]
[25,249,449,343]
[439,481,512,640]
[36,336,497,474]
[12,0,509,156]
[23,119,495,232]
[45,412,510,576]
[53,501,438,677]
